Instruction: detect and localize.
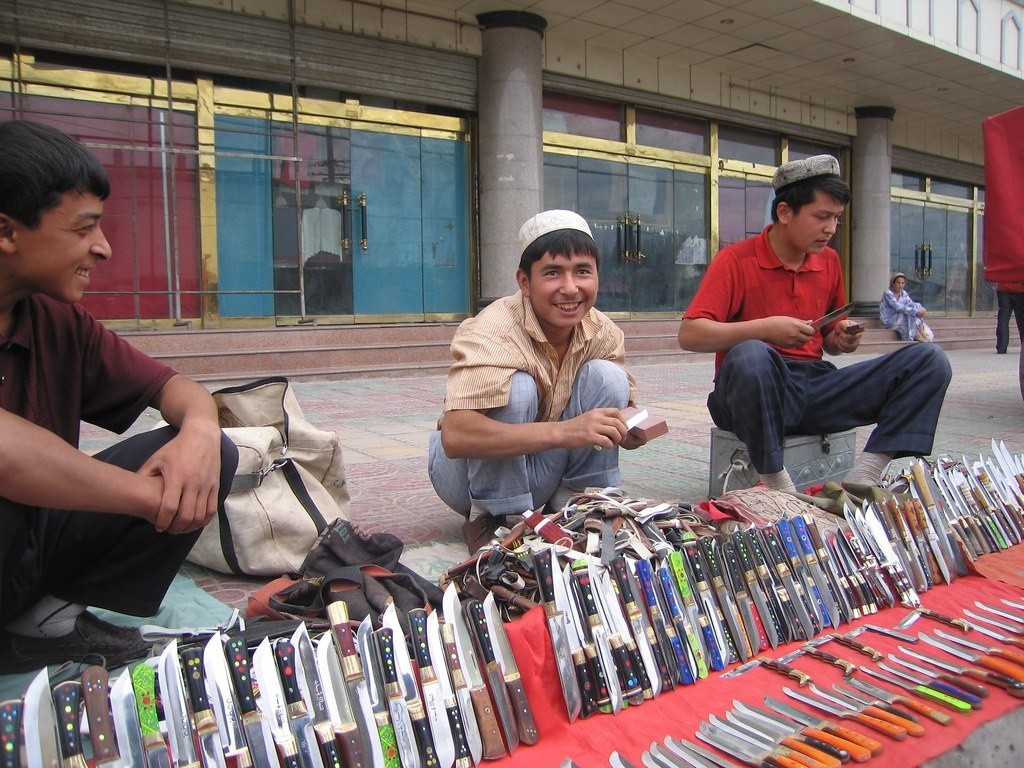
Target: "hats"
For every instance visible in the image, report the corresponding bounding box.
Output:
[518,209,595,268]
[773,154,840,195]
[891,272,904,282]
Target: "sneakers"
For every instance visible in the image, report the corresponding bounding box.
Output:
[462,509,511,558]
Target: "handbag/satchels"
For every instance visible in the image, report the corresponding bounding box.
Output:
[153,374,352,577]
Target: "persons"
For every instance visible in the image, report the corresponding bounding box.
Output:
[428,209,648,557]
[0,119,239,677]
[995,282,1024,354]
[879,272,929,342]
[678,154,952,492]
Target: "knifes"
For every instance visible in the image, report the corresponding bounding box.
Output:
[593,407,649,454]
[806,303,860,332]
[0,441,1023,768]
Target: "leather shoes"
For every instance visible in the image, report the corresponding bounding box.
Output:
[0,611,156,675]
